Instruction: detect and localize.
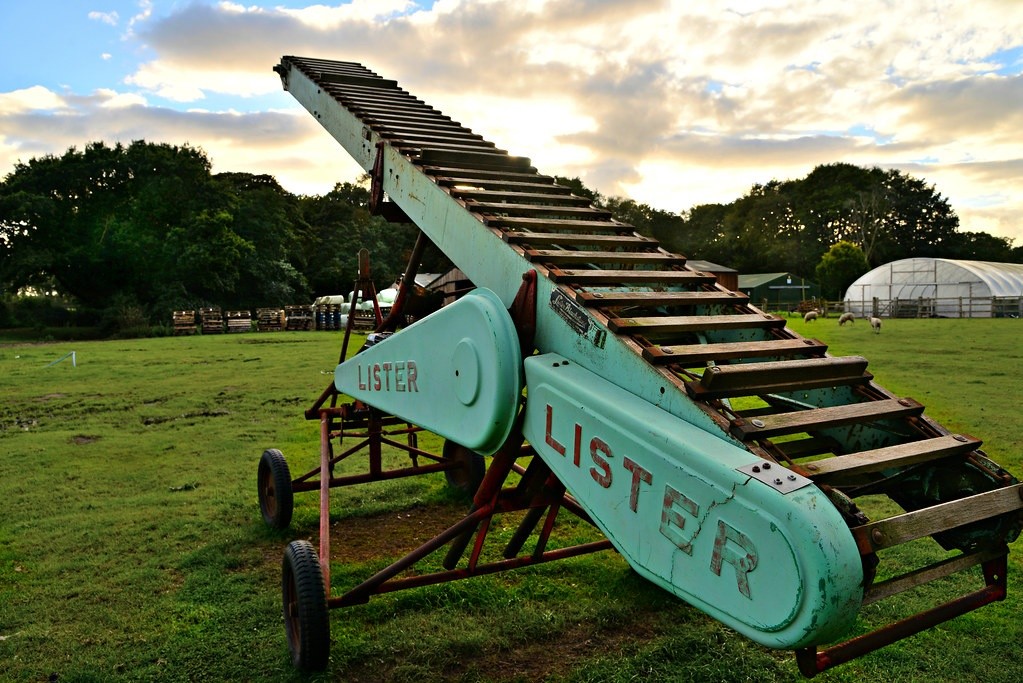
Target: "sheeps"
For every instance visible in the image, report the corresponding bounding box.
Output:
[839,312,854,327]
[866,315,881,333]
[804,311,818,323]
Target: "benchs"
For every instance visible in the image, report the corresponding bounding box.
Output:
[228,319,252,334]
